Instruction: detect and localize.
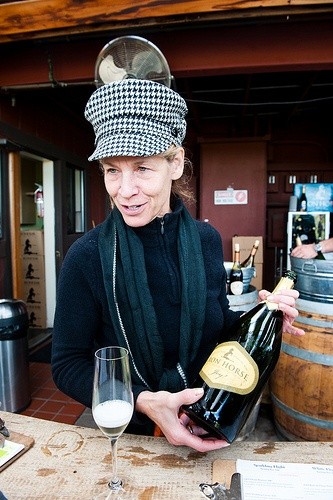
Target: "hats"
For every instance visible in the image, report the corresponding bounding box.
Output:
[84,79,189,162]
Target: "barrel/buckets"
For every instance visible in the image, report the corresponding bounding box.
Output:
[223,261,256,294]
[269,298,333,442]
[289,251,333,304]
[0,298,32,414]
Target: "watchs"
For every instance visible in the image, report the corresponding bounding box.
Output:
[314,243,322,255]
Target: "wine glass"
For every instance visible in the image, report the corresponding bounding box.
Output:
[92,346,145,500]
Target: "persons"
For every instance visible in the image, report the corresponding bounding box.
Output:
[52,79,306,452]
[291,238,333,260]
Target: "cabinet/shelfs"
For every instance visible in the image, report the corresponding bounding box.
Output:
[265,163,333,291]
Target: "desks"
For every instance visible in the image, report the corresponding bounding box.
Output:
[0,410,333,500]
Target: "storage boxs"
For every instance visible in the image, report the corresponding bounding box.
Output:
[21,228,47,328]
[232,236,263,290]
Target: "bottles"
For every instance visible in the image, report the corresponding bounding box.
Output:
[300,185,307,212]
[240,240,259,268]
[229,243,244,295]
[180,267,297,445]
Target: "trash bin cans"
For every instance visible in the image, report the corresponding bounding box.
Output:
[0,298,32,413]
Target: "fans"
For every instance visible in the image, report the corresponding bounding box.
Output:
[94,36,171,88]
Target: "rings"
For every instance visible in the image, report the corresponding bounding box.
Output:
[188,425,194,436]
